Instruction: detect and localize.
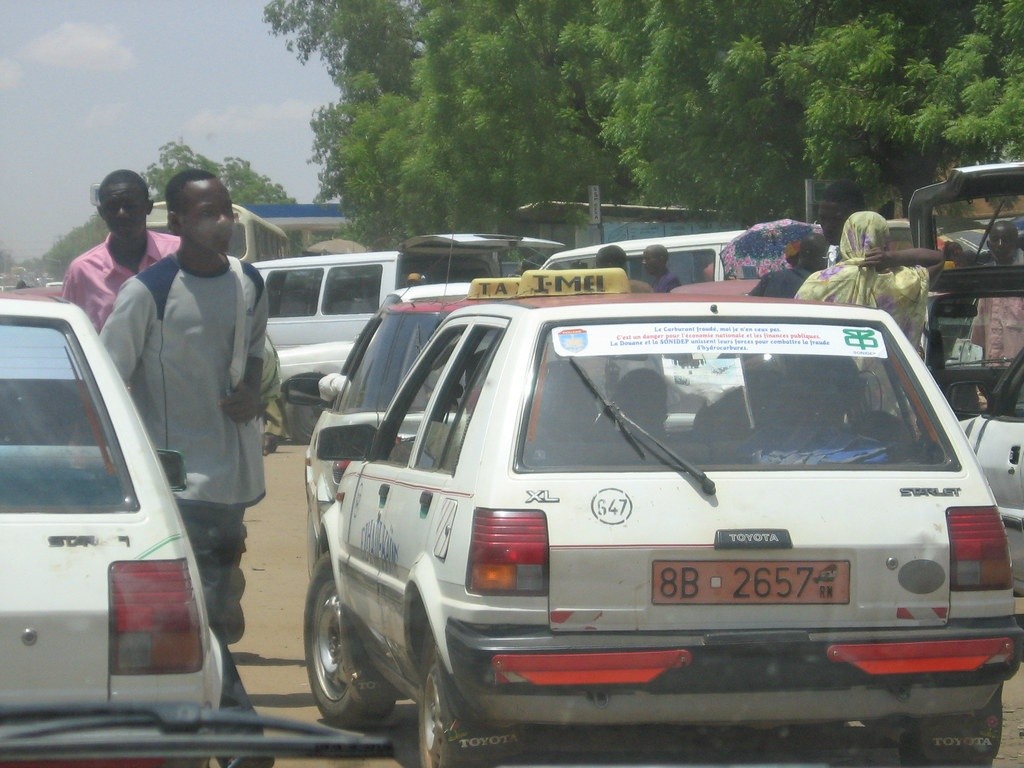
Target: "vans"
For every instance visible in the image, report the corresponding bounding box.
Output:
[253,233,564,441]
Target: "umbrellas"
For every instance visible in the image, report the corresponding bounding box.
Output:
[718,218,824,280]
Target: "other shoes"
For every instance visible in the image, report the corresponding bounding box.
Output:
[225,754,274,768]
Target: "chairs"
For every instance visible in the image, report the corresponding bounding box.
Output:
[610,370,668,447]
[535,361,599,443]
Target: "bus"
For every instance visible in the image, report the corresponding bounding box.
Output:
[148,202,292,265]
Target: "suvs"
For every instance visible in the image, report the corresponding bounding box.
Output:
[543,226,829,291]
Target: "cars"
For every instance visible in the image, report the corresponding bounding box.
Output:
[946,331,1024,597]
[886,159,1024,400]
[0,298,221,768]
[304,268,1024,768]
[0,266,65,297]
[298,275,745,539]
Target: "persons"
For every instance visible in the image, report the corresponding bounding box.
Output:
[747,210,945,349]
[943,221,1024,366]
[60,168,276,768]
[406,273,420,287]
[596,245,682,293]
